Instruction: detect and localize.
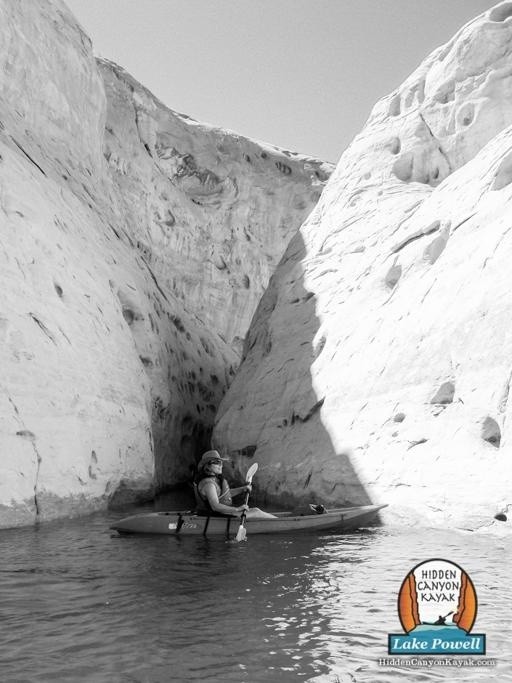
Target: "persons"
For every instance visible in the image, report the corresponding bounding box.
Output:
[197,450,280,518]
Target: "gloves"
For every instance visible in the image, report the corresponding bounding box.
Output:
[111,502,390,539]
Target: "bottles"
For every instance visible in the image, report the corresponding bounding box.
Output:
[197,449,230,471]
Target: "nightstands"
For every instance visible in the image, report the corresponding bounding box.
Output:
[235,463,258,541]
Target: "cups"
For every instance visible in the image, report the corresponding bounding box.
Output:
[210,459,222,465]
